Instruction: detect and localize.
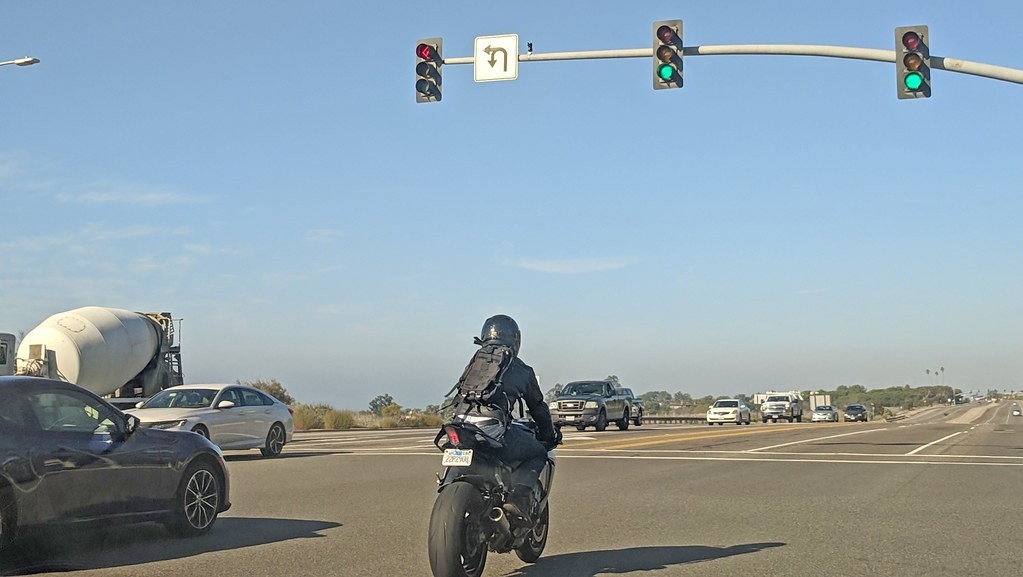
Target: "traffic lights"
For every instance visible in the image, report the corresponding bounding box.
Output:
[415,37,443,103]
[895,25,931,99]
[652,19,684,89]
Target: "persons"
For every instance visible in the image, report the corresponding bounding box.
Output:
[444,316,556,525]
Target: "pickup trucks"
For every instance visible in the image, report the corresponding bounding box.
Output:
[614,386,644,426]
[550,380,633,431]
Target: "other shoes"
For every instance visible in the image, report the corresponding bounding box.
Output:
[504,487,533,518]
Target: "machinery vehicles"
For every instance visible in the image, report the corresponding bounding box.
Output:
[14,306,184,410]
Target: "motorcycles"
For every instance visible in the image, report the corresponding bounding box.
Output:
[427,416,586,577]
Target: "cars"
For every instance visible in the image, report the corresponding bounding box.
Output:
[972,396,1021,416]
[0,374,232,553]
[843,404,868,422]
[100,382,295,458]
[811,406,839,422]
[707,398,752,426]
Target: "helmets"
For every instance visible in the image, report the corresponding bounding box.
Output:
[483,314,518,345]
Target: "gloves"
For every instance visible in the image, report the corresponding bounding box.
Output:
[535,425,564,452]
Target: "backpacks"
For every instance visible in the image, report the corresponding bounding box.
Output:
[442,347,515,407]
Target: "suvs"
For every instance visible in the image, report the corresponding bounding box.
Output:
[760,394,802,423]
